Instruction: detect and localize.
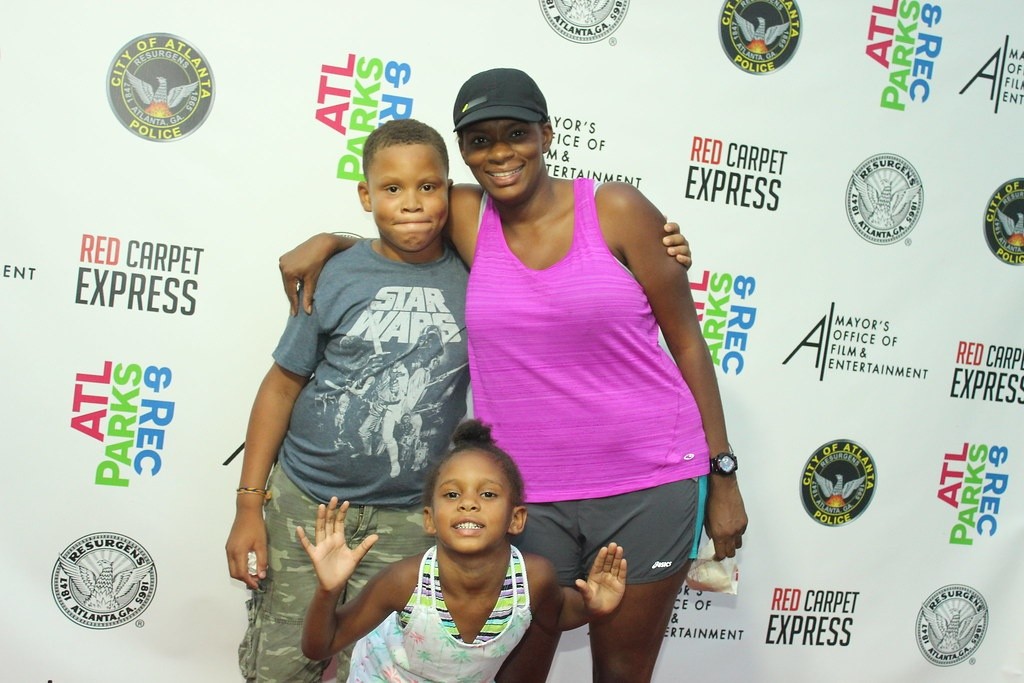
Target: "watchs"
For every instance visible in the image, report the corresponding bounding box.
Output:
[711,453,738,473]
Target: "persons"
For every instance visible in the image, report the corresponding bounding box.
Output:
[225,119,693,683]
[296,419,627,683]
[278,70,749,683]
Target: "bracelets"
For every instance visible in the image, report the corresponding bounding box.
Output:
[235,487,273,500]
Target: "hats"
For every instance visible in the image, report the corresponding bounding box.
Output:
[453,68,547,133]
